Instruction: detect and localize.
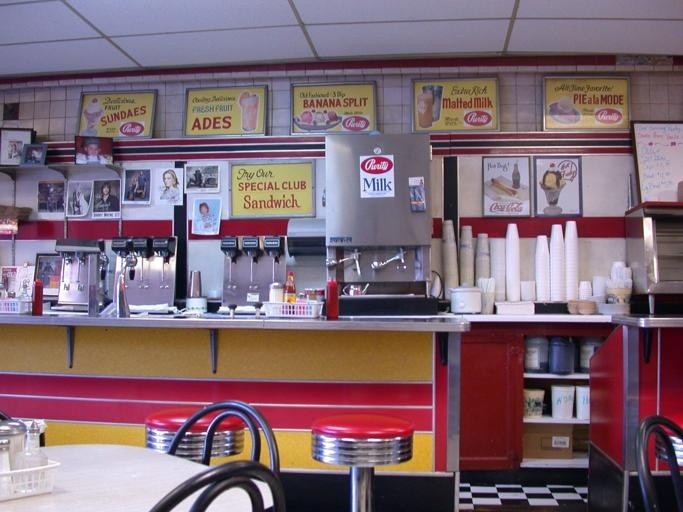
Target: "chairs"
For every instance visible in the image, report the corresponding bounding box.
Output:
[635,416,683,510]
[145,399,286,512]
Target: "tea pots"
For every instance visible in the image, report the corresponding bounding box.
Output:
[342,282,369,296]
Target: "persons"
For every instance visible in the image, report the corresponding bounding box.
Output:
[136,171,149,190]
[96,182,121,213]
[127,174,145,199]
[195,201,216,226]
[159,169,177,203]
[7,141,22,161]
[74,140,111,165]
[40,260,53,274]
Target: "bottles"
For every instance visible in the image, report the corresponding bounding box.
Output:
[267,283,284,303]
[189,271,201,297]
[510,162,520,189]
[283,271,297,303]
[31,278,43,316]
[325,276,339,320]
[14,420,49,485]
[0,438,13,495]
[0,418,27,471]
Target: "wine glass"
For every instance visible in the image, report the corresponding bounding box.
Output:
[540,184,567,215]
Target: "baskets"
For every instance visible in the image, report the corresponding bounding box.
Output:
[0,459,61,502]
[261,300,325,319]
[0,299,26,314]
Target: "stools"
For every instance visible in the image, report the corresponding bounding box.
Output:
[309,412,414,511]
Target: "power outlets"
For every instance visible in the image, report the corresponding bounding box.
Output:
[460,323,680,484]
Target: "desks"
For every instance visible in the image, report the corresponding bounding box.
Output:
[1,443,275,512]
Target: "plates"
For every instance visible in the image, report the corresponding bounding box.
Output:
[292,116,343,131]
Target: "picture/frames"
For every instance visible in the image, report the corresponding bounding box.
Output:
[74,135,114,166]
[1,128,49,169]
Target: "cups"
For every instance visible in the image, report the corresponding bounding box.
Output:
[578,300,596,315]
[416,93,433,129]
[239,95,258,131]
[575,384,590,420]
[422,85,442,122]
[439,218,634,302]
[481,293,495,314]
[549,383,575,417]
[522,388,545,417]
[567,300,579,314]
[449,283,482,314]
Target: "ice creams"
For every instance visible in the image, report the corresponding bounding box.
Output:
[541,163,564,189]
[84,97,103,129]
